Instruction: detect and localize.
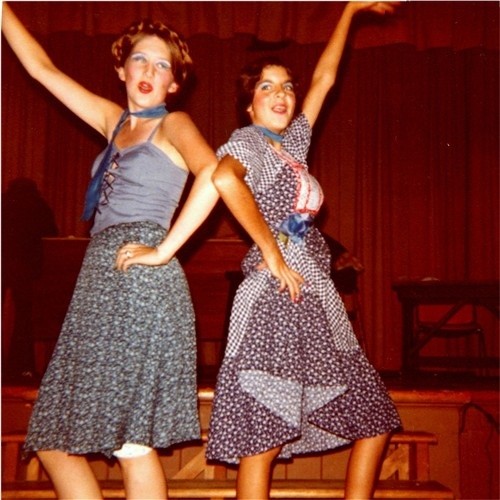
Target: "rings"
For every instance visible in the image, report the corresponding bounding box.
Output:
[125,250,131,259]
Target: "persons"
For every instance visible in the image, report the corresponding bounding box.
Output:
[210,0,403,499]
[1,1,220,500]
